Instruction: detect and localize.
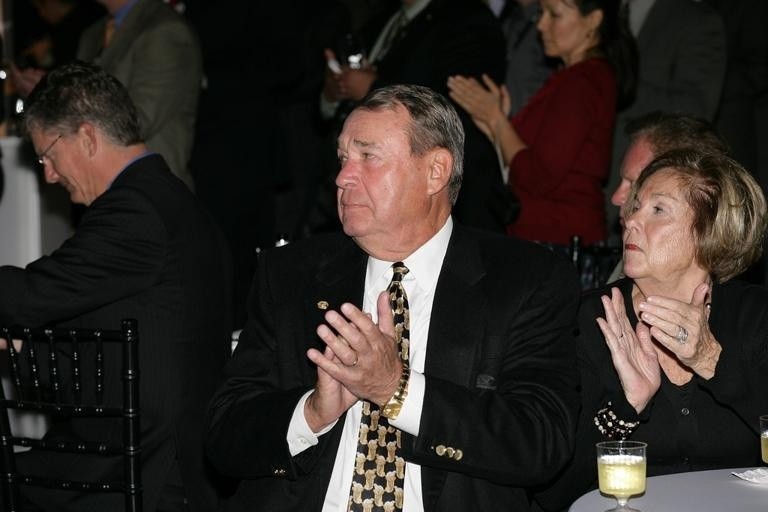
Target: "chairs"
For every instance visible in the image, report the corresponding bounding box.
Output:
[0,314,144,512]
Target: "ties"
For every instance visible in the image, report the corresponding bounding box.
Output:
[345,262,410,512]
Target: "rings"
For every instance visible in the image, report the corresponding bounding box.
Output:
[617,334,623,340]
[345,352,359,368]
[676,326,689,344]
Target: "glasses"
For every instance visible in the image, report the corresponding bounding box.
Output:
[36,134,61,166]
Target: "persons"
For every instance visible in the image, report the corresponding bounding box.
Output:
[0,0,766,286]
[0,62,237,508]
[203,80,580,512]
[526,145,766,511]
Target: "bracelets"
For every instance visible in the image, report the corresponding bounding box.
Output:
[378,365,410,421]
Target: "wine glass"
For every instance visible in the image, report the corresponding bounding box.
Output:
[595,442,646,512]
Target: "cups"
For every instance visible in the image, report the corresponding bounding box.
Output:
[759,415,768,464]
